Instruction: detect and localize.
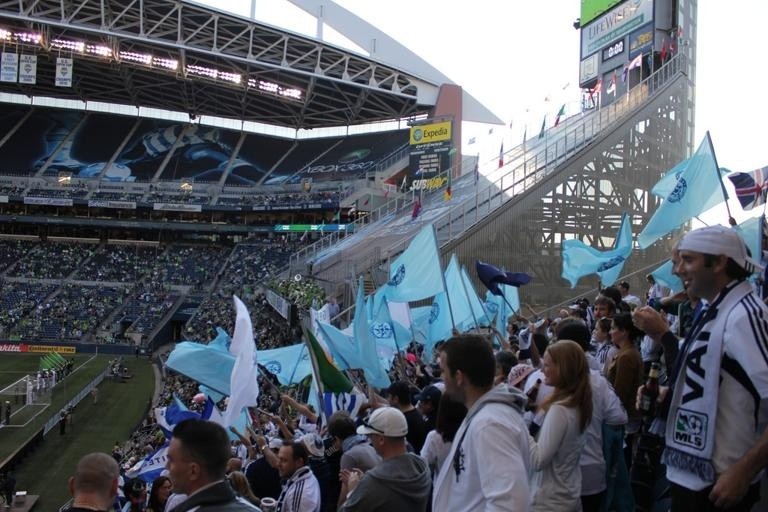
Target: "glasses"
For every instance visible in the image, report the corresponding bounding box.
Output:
[601,315,609,328]
[363,415,388,435]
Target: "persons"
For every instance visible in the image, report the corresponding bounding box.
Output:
[60,410,66,434]
[578,299,587,308]
[0,212,330,226]
[4,400,11,424]
[646,274,660,305]
[136,347,139,361]
[112,442,119,451]
[635,225,768,511]
[148,441,154,453]
[112,450,115,455]
[493,329,519,350]
[432,337,530,512]
[166,418,262,512]
[414,385,441,430]
[131,447,135,456]
[114,448,120,461]
[123,374,210,448]
[122,478,147,512]
[598,287,630,313]
[22,387,27,404]
[507,336,517,340]
[147,476,172,512]
[4,472,15,509]
[142,442,149,456]
[63,452,120,512]
[134,440,140,456]
[1,181,344,207]
[381,383,431,454]
[508,364,537,390]
[123,368,128,377]
[509,323,517,334]
[338,407,431,512]
[602,313,640,512]
[524,318,628,512]
[121,447,124,457]
[629,238,699,512]
[14,385,19,402]
[583,297,594,331]
[568,305,579,314]
[24,382,28,389]
[236,440,247,469]
[493,351,518,384]
[230,439,238,456]
[594,295,616,319]
[255,435,321,512]
[618,282,641,307]
[647,289,699,337]
[92,386,99,404]
[110,359,117,373]
[510,344,519,353]
[228,471,270,512]
[226,426,255,475]
[592,317,611,376]
[529,340,593,512]
[528,322,548,370]
[558,309,569,319]
[420,391,468,486]
[254,363,321,437]
[516,315,547,358]
[328,411,382,510]
[524,301,552,325]
[1,235,304,347]
[575,308,587,321]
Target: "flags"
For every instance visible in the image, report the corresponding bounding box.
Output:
[670,31,678,53]
[373,222,446,318]
[222,296,258,427]
[316,320,363,370]
[353,276,392,390]
[369,296,398,348]
[461,265,486,332]
[637,132,729,251]
[412,197,422,220]
[364,195,372,205]
[539,117,546,138]
[201,395,227,428]
[303,326,355,394]
[377,320,411,348]
[347,204,356,215]
[555,105,566,126]
[445,169,453,201]
[300,230,308,241]
[423,252,473,361]
[257,344,312,386]
[473,155,480,185]
[333,212,339,222]
[485,285,506,348]
[497,283,520,317]
[416,167,423,175]
[595,81,604,92]
[124,445,169,481]
[154,408,174,443]
[198,327,232,404]
[401,175,407,192]
[607,70,617,94]
[165,398,197,423]
[621,64,630,83]
[383,189,388,196]
[318,221,324,231]
[561,213,632,287]
[728,168,768,209]
[651,215,762,293]
[499,144,504,167]
[324,393,361,422]
[447,147,454,156]
[649,156,731,219]
[648,46,653,71]
[476,259,532,297]
[660,38,668,59]
[407,306,433,338]
[627,53,643,69]
[164,342,235,397]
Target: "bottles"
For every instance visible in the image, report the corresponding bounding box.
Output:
[638,361,661,418]
[526,378,542,408]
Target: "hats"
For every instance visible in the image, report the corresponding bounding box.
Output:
[306,433,326,459]
[571,304,580,309]
[576,299,587,306]
[414,386,443,404]
[271,438,283,449]
[517,319,545,351]
[508,364,536,385]
[433,382,447,391]
[679,227,766,275]
[357,408,410,437]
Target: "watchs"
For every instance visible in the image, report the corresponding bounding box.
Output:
[262,444,268,450]
